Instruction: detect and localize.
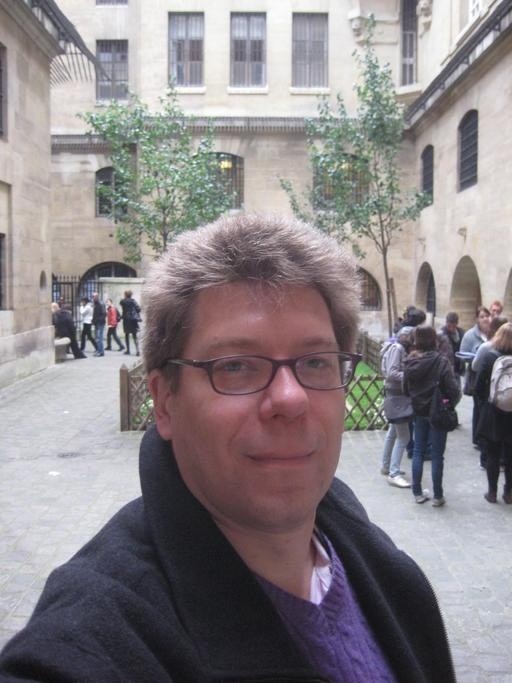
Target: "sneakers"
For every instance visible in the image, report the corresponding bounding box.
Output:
[416,489,432,504]
[431,496,448,507]
[408,448,443,462]
[387,474,411,488]
[483,490,511,506]
[380,466,406,475]
[68,342,140,358]
[473,442,506,472]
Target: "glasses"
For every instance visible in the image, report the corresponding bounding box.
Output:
[163,349,364,394]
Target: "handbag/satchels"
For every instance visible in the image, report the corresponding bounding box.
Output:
[429,358,459,431]
[383,380,415,424]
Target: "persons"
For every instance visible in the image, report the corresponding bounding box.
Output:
[1,200,460,681]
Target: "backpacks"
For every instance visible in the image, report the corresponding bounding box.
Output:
[487,356,512,413]
